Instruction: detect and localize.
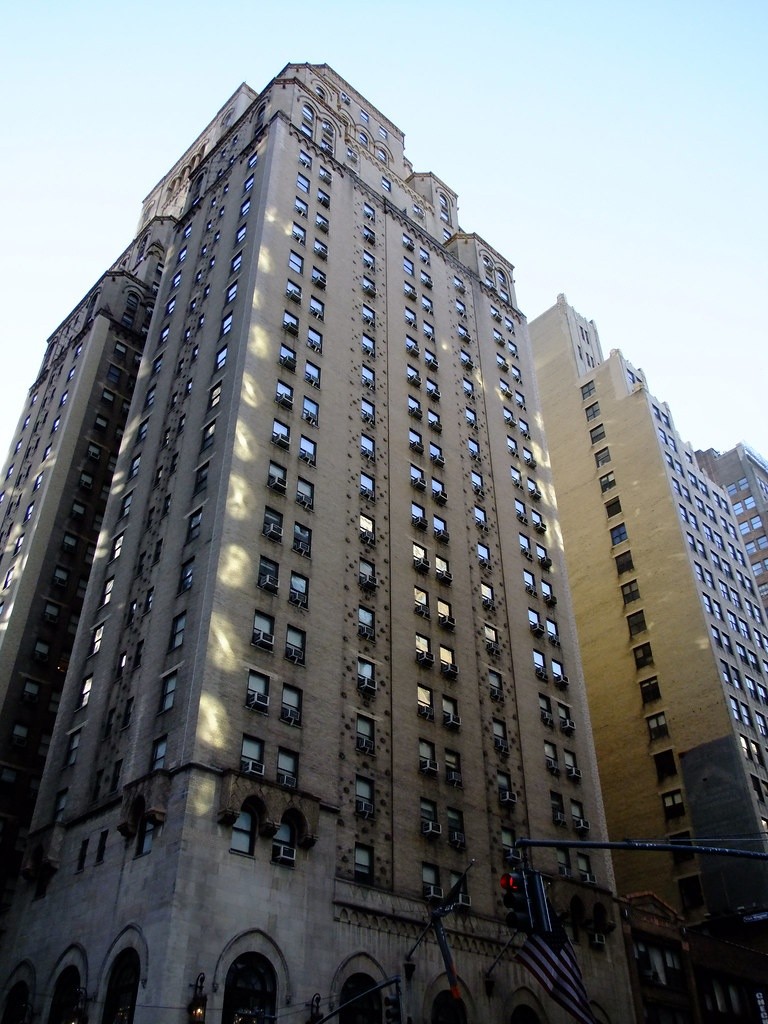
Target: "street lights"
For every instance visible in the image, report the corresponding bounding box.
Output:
[311,993,324,1024]
[68,987,89,1024]
[188,973,208,1024]
[15,1000,34,1024]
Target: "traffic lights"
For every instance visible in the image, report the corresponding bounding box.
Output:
[384,994,401,1024]
[500,868,536,932]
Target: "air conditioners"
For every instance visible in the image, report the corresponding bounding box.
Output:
[280,775,296,788]
[560,867,606,946]
[453,893,473,908]
[243,761,266,777]
[273,433,291,448]
[299,496,312,507]
[288,649,304,662]
[274,845,297,865]
[301,451,315,464]
[250,692,270,710]
[260,574,280,592]
[425,886,445,901]
[269,476,288,493]
[254,632,275,649]
[283,709,300,724]
[296,541,310,555]
[291,593,308,607]
[357,432,591,861]
[264,524,283,540]
[279,96,526,431]
